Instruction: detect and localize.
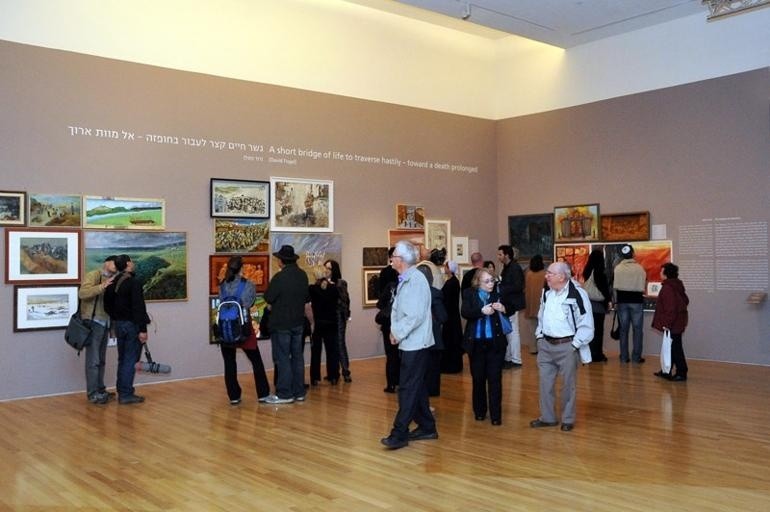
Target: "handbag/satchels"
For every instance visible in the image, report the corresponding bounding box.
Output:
[659,327,675,376]
[609,310,621,340]
[61,309,101,358]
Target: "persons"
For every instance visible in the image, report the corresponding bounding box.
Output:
[102,255,152,404]
[78,256,117,402]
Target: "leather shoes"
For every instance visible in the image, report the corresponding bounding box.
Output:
[383,382,396,395]
[474,414,487,422]
[528,417,559,429]
[560,423,574,433]
[407,428,441,441]
[490,419,502,427]
[378,432,410,450]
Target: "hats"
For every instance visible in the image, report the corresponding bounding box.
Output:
[447,260,457,273]
[279,242,296,262]
[620,243,634,257]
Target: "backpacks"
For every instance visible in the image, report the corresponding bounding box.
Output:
[212,276,254,348]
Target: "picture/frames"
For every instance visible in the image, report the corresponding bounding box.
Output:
[1,190,191,334]
[359,200,479,309]
[504,202,676,315]
[206,174,338,346]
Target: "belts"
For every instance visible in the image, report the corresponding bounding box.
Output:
[541,332,574,345]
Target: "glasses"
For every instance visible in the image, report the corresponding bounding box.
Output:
[390,252,401,260]
[543,268,560,278]
[323,264,333,273]
[484,277,495,286]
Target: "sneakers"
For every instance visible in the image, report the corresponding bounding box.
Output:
[85,389,117,406]
[118,393,146,405]
[343,374,353,385]
[228,397,242,407]
[322,374,342,386]
[297,394,306,404]
[309,378,320,387]
[618,355,631,364]
[502,359,524,370]
[258,392,296,405]
[652,370,689,383]
[631,357,645,363]
